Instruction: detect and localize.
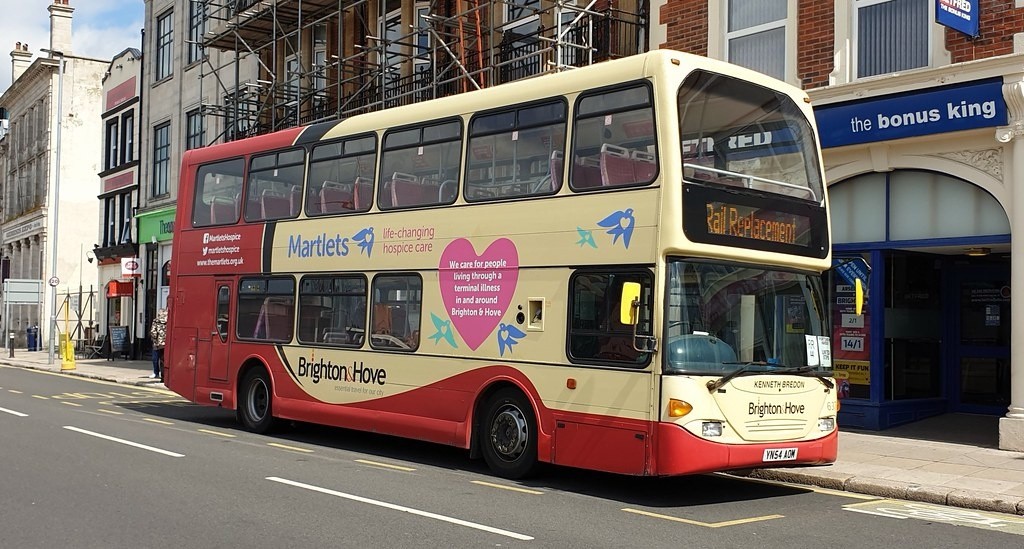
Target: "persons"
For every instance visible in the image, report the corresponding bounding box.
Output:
[150,309,166,383]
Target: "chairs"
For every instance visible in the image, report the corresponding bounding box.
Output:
[260,189,290,218]
[353,176,382,210]
[290,184,319,216]
[84,335,107,359]
[472,188,496,198]
[319,181,352,213]
[498,185,527,196]
[579,157,602,186]
[264,297,293,340]
[383,181,392,207]
[631,150,655,182]
[314,309,355,342]
[420,177,450,203]
[550,150,585,191]
[210,196,237,223]
[694,165,745,187]
[234,192,261,221]
[388,302,411,338]
[391,171,424,207]
[599,143,637,186]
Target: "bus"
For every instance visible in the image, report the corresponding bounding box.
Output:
[165,51,873,478]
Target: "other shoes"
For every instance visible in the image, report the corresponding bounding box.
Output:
[154,373,159,379]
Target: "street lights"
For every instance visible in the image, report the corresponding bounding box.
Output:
[39,46,64,365]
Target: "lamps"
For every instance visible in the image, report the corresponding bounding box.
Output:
[963,248,992,256]
[85,250,95,264]
[109,248,122,261]
[94,248,106,262]
